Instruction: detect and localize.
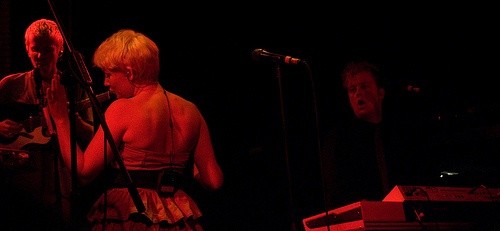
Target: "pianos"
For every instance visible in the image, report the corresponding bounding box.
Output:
[302,184,500,231]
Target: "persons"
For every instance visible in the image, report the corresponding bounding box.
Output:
[0,19,93,231]
[46,29,224,231]
[327,60,442,202]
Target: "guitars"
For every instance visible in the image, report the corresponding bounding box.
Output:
[0,90,116,160]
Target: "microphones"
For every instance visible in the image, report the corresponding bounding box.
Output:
[32,67,47,107]
[251,48,302,65]
